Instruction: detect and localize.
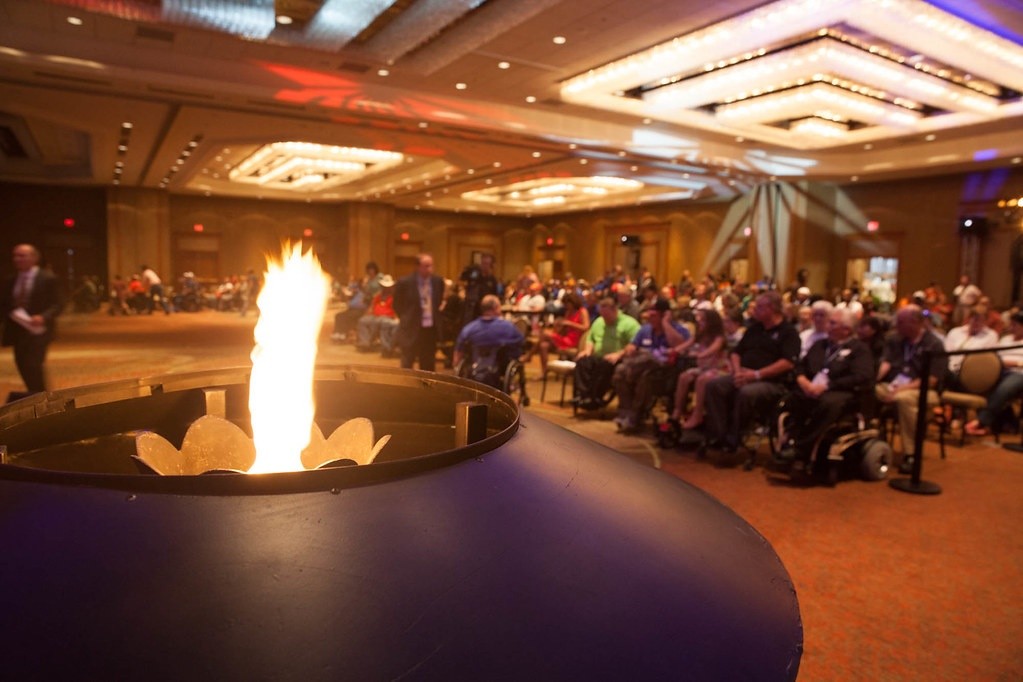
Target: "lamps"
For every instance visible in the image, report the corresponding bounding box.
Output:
[461,176,643,212]
[227,140,406,192]
[564,0,1023,152]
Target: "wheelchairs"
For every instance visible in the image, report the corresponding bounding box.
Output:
[334,303,452,369]
[109,290,250,313]
[575,344,893,485]
[455,341,530,407]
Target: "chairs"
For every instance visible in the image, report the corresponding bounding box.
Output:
[938,352,1006,458]
[880,374,949,465]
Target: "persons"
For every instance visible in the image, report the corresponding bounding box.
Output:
[329,254,505,370]
[80,275,95,311]
[110,265,258,317]
[0,245,60,394]
[452,295,526,388]
[505,264,1023,489]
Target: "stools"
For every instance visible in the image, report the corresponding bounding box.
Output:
[540,360,576,408]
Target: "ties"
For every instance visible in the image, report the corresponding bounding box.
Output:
[423,278,431,313]
[19,273,28,303]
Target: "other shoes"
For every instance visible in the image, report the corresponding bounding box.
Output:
[616,416,639,430]
[571,394,596,409]
[965,419,989,435]
[900,455,916,475]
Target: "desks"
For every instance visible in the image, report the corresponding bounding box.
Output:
[500,305,561,325]
